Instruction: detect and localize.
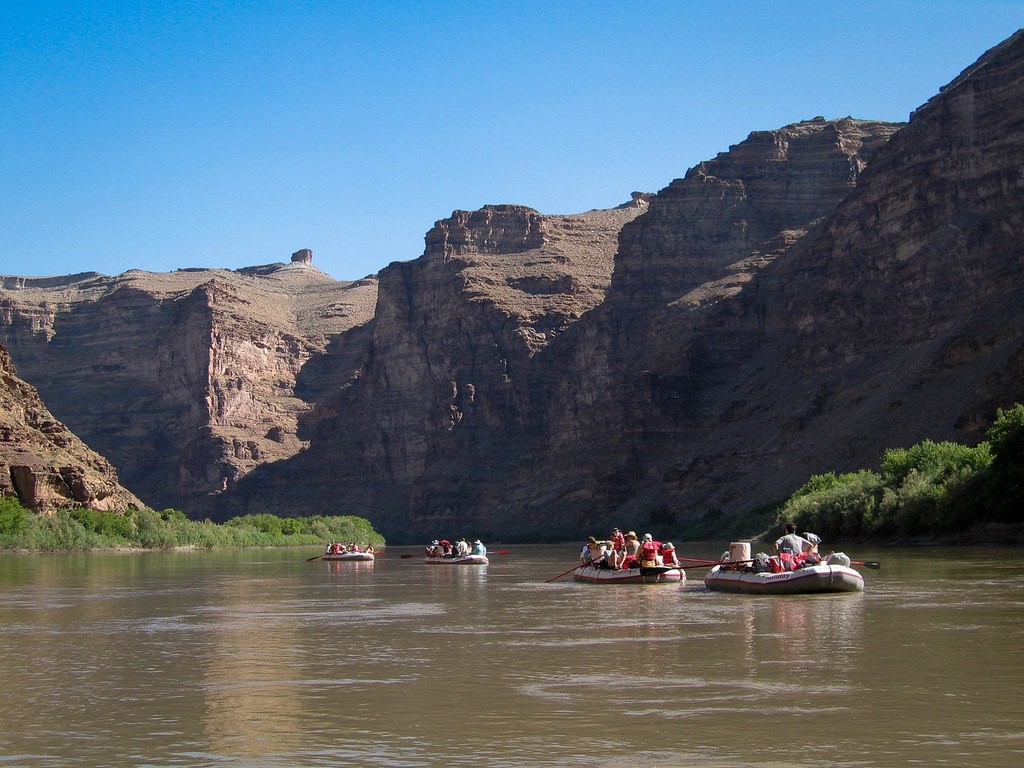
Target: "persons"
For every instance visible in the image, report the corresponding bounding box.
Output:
[661,542,681,566]
[579,528,628,570]
[430,538,472,557]
[774,523,814,555]
[635,533,661,581]
[469,539,487,556]
[326,540,358,553]
[624,530,641,569]
[365,544,374,554]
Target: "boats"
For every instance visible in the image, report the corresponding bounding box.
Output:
[704,566,865,593]
[322,552,375,561]
[573,564,680,583]
[424,554,490,564]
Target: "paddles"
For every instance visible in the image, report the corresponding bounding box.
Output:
[487,549,513,555]
[373,551,385,555]
[639,555,778,577]
[543,554,604,583]
[307,554,325,562]
[400,554,427,559]
[850,559,882,571]
[677,557,716,563]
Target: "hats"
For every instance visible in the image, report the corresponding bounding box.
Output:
[474,539,482,544]
[431,540,439,544]
[662,541,676,548]
[623,531,637,539]
[642,533,653,541]
[441,540,450,546]
[611,527,620,533]
